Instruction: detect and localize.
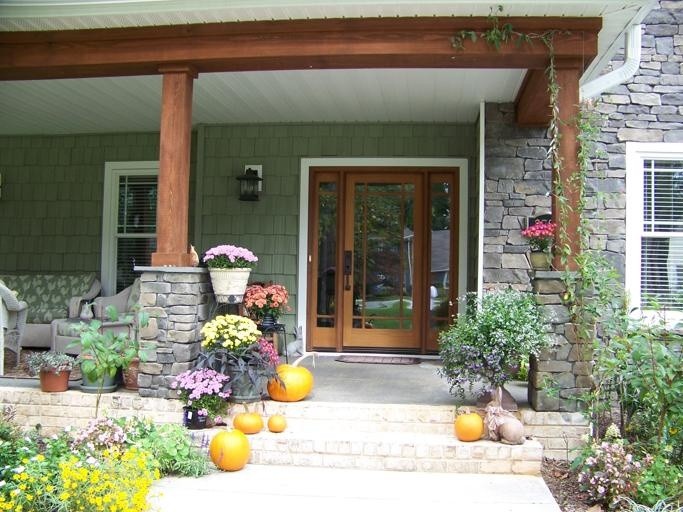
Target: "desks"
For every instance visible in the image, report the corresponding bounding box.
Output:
[256,323,289,364]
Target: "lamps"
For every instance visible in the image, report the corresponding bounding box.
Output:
[234,164,264,203]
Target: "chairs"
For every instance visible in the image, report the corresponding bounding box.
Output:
[0,271,139,376]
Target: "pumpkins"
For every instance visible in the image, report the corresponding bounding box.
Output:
[455,412,483,441]
[210,428,249,471]
[267,412,287,433]
[266,351,319,401]
[232,402,263,434]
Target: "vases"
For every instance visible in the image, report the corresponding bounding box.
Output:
[474,377,519,412]
[207,268,251,304]
[529,251,551,271]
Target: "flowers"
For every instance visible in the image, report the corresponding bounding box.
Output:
[171,313,279,429]
[433,280,564,404]
[521,218,557,251]
[200,243,258,267]
[244,277,290,313]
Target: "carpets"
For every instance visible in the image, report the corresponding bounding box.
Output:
[4,345,81,379]
[333,354,425,366]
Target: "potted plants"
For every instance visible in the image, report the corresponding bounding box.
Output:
[38,284,149,392]
